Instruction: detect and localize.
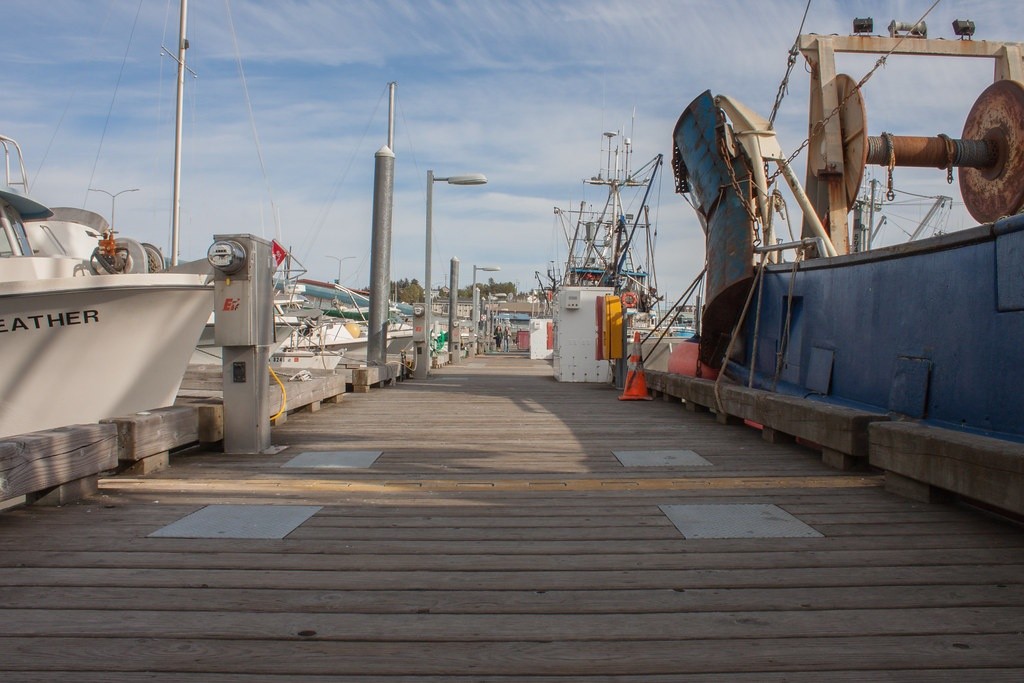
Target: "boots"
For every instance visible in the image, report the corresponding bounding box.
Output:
[496,347,500,352]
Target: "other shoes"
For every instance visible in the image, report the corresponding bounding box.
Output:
[507,351,510,352]
[503,351,506,352]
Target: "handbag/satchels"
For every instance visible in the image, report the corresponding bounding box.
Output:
[493,335,497,339]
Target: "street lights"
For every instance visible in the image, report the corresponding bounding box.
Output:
[87,187,139,242]
[471,263,501,358]
[426,173,487,372]
[487,292,511,354]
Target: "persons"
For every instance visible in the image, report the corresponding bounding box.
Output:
[493,326,511,352]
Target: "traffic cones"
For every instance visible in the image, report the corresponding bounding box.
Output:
[616,330,652,400]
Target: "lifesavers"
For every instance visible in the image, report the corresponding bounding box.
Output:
[622,291,637,308]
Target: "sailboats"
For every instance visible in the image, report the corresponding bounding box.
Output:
[81,0,300,364]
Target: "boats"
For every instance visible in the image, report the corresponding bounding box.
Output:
[280,268,448,367]
[0,132,222,441]
[548,124,718,380]
